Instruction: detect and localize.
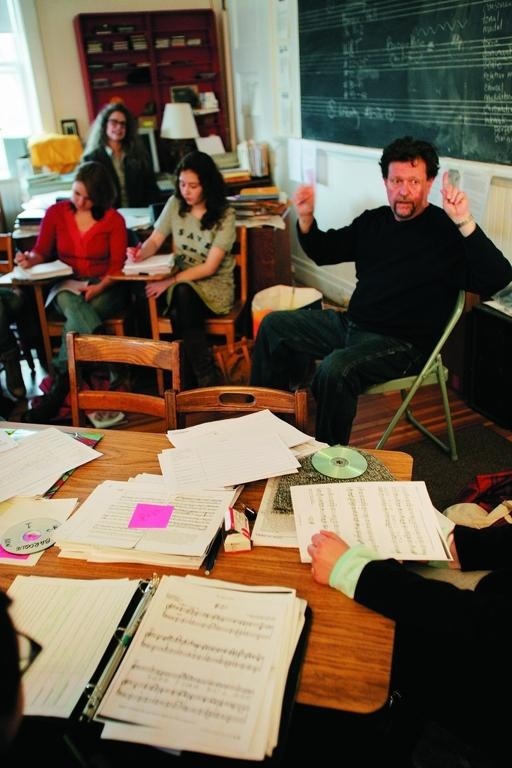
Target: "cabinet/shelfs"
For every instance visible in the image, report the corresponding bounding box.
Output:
[469,303,511,430]
[74,9,236,172]
[232,202,291,290]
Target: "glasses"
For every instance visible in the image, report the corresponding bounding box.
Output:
[16,630,42,678]
[110,119,126,129]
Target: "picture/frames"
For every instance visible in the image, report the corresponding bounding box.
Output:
[61,121,79,135]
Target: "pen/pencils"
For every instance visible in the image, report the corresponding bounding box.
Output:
[15,247,27,259]
[205,528,223,570]
[132,242,141,256]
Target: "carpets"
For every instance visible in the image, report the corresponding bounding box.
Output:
[394,424,512,510]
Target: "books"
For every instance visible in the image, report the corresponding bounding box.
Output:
[226,185,288,228]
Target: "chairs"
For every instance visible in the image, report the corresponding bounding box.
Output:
[0,233,24,337]
[146,225,250,397]
[30,228,129,378]
[362,288,465,461]
[63,332,182,436]
[162,385,310,439]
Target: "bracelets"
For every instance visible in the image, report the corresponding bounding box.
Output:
[455,214,475,228]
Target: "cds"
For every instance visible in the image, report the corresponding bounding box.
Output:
[311,446,369,480]
[1,517,62,554]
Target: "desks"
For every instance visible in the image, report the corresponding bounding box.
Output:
[13,170,273,339]
[0,421,414,768]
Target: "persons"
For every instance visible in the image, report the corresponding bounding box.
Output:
[307,528,511,768]
[133,150,237,388]
[80,101,161,207]
[14,161,128,422]
[0,590,42,767]
[251,135,512,446]
[0,290,26,399]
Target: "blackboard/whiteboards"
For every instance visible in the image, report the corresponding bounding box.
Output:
[297,0,512,166]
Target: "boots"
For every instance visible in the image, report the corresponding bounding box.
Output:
[0,349,27,399]
[91,324,129,391]
[20,364,69,422]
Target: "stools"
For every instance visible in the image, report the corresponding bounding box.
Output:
[251,283,325,342]
